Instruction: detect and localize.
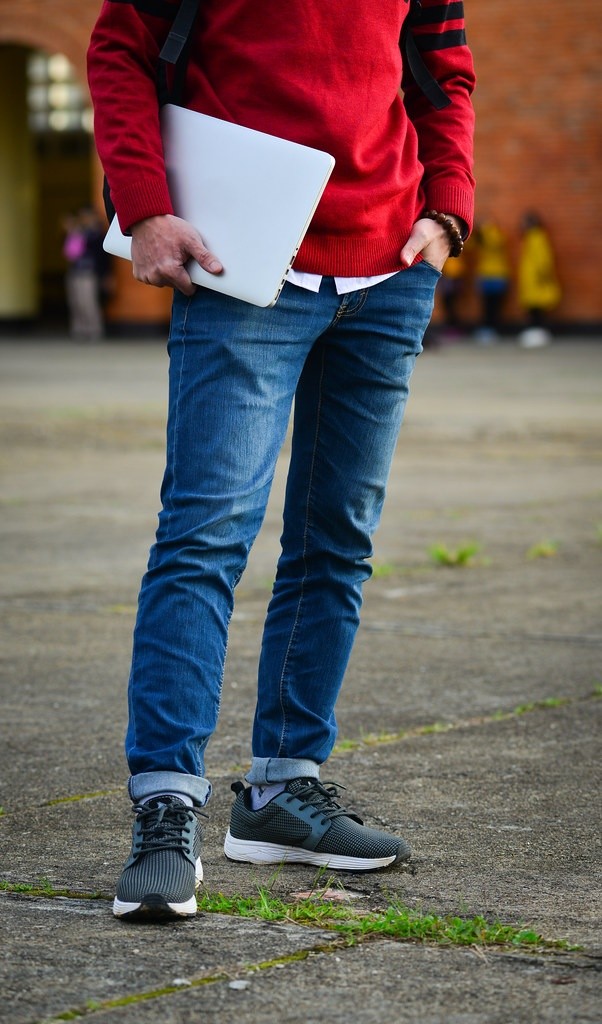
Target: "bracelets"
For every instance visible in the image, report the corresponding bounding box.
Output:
[424,210,464,259]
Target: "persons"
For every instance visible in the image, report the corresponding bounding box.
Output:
[54,203,114,341]
[82,0,478,922]
[421,207,563,349]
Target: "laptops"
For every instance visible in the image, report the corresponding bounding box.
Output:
[102,105,336,308]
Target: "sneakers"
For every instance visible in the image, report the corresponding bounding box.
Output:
[112,794,210,923]
[224,777,412,874]
